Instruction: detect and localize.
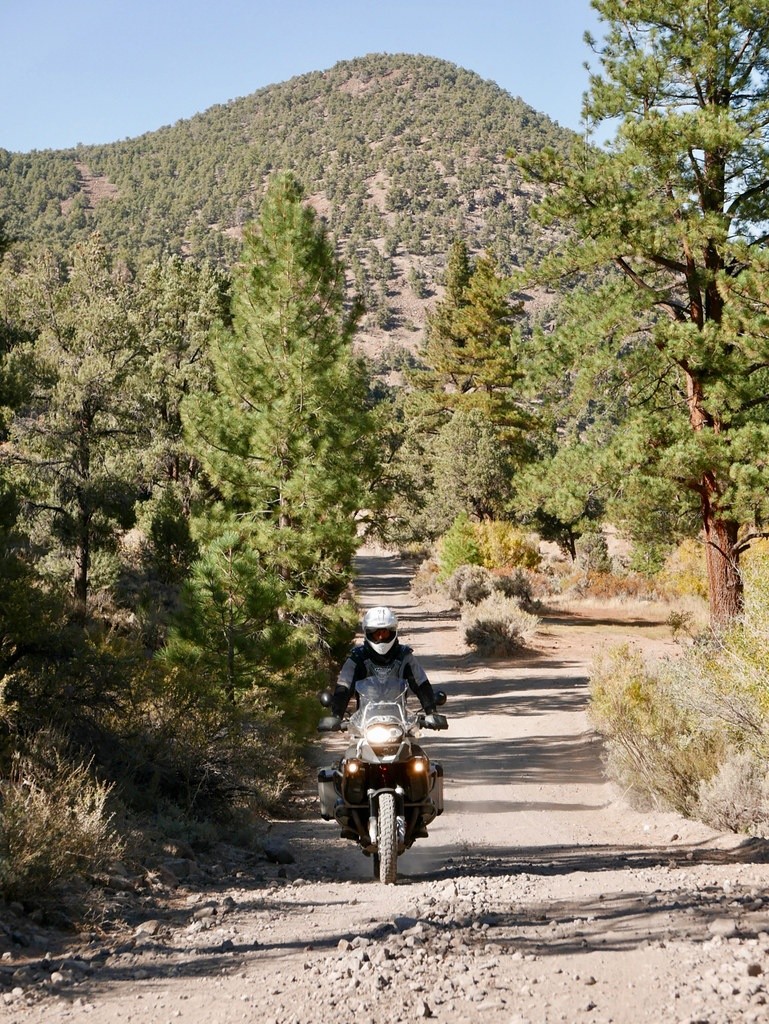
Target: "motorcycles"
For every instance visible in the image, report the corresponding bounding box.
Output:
[315,690,451,884]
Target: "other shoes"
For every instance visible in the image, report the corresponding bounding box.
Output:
[415,823,429,837]
[341,822,359,841]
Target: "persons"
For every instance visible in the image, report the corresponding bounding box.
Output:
[320,607,443,837]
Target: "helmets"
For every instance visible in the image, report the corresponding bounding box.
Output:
[362,606,399,654]
